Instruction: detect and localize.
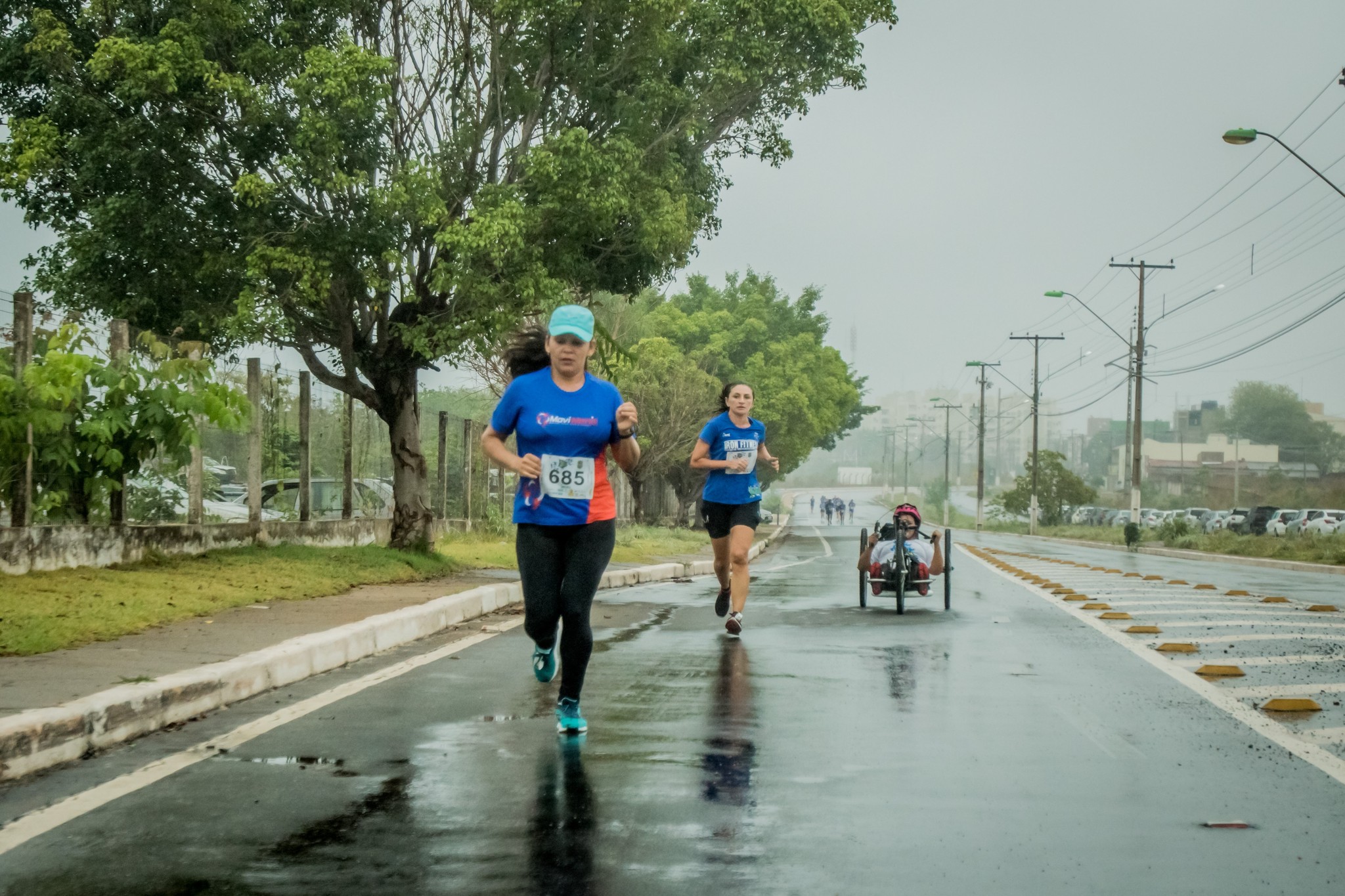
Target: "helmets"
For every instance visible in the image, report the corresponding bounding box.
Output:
[893,502,921,529]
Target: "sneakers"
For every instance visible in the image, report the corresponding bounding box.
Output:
[532,622,562,682]
[725,611,743,636]
[715,578,731,617]
[556,697,587,732]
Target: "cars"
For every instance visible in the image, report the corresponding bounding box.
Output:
[96,455,396,524]
[1060,503,1345,538]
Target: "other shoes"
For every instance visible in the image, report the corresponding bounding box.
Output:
[917,563,930,596]
[870,562,882,596]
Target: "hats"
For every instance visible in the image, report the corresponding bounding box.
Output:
[548,305,595,342]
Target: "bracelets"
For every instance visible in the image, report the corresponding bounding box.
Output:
[619,429,634,439]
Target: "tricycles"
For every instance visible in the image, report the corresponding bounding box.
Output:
[859,507,955,615]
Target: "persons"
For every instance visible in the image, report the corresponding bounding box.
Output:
[857,505,944,596]
[690,379,779,637]
[480,304,643,734]
[809,495,855,525]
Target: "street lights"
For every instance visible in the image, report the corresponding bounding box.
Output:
[929,396,985,530]
[905,417,949,527]
[1044,290,1145,527]
[966,360,1039,536]
